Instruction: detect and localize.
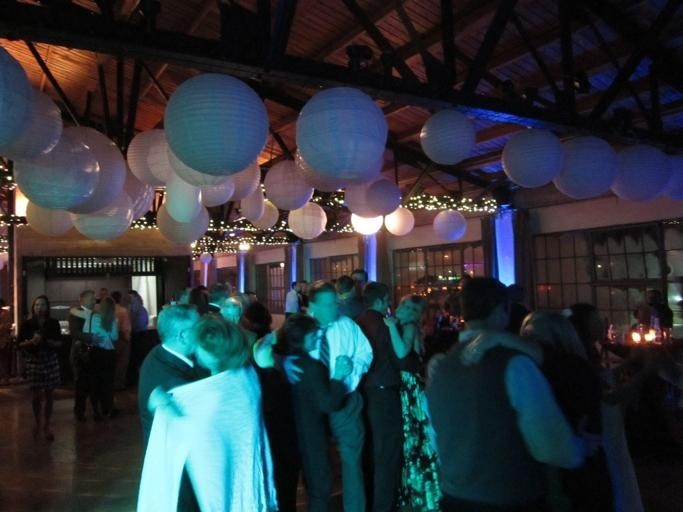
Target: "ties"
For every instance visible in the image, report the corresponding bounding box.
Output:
[314,325,333,383]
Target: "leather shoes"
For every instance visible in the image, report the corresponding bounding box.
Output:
[30,405,122,442]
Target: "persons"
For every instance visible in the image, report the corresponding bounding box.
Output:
[67,268,682,512]
[15,294,65,440]
